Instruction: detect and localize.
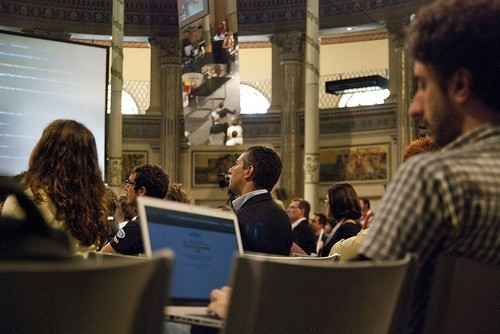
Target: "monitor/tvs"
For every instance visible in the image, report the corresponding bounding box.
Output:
[178,0,208,29]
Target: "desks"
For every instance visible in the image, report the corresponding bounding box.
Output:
[185,76,231,119]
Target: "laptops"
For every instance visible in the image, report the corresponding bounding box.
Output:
[136,195,246,328]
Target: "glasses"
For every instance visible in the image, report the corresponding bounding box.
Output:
[322,196,331,204]
[310,219,317,224]
[125,178,137,187]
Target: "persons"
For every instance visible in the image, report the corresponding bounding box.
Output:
[181,31,194,62]
[209,102,243,146]
[329,133,440,263]
[99,163,169,258]
[272,181,375,258]
[216,205,232,213]
[206,0,500,334]
[0,171,29,212]
[189,147,292,334]
[104,187,132,228]
[163,183,194,204]
[2,119,110,258]
[211,21,234,74]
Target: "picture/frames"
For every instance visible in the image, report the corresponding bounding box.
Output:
[319,141,392,185]
[191,150,245,189]
[122,150,148,182]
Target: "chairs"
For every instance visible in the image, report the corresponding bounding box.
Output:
[417,250,500,334]
[222,252,413,334]
[0,248,175,334]
